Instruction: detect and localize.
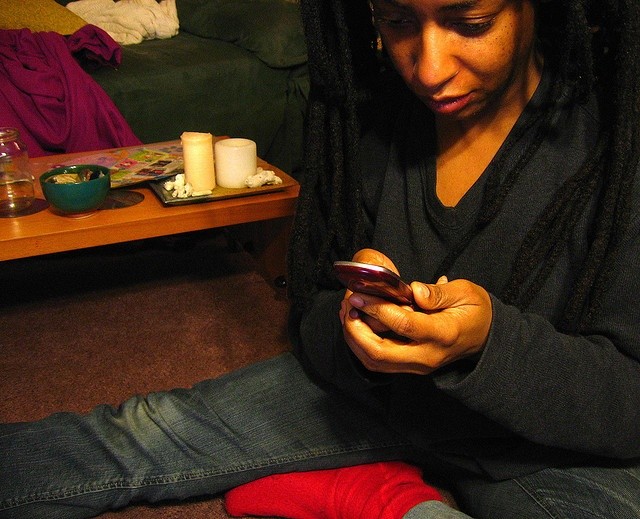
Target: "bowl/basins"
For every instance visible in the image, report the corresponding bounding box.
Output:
[39,164,111,218]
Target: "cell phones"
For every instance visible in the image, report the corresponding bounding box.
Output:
[333,260,413,305]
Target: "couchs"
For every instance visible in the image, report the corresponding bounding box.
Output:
[0,0,309,298]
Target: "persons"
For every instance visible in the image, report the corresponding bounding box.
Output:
[1,1,639,518]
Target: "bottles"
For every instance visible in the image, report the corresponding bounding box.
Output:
[0,127,35,215]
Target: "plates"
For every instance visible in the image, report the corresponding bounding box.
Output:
[147,156,298,206]
